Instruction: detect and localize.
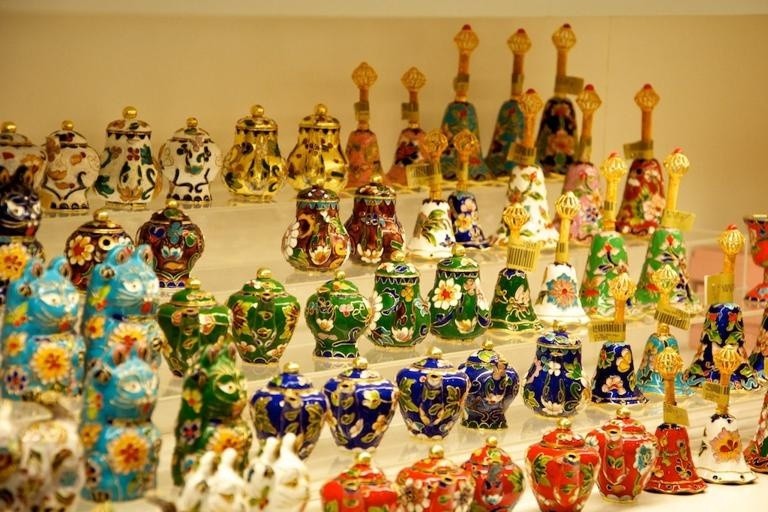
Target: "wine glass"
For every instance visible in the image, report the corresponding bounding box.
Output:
[742,213,768,307]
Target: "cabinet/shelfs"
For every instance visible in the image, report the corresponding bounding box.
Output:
[33,176,767,512]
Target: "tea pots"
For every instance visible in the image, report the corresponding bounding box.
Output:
[0,101,662,512]
